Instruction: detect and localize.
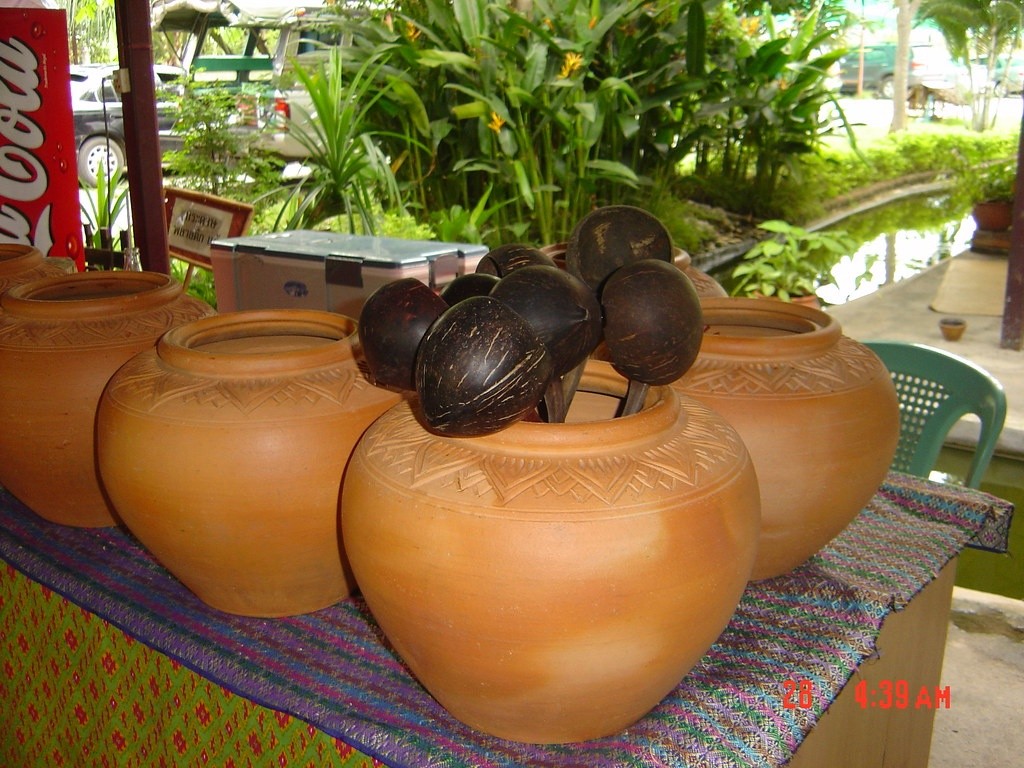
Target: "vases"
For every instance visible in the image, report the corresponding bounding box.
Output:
[0,268,213,530]
[534,243,732,298]
[939,319,966,340]
[94,310,412,625]
[336,364,764,745]
[0,240,77,294]
[600,296,901,584]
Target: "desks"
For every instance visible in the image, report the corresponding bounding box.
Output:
[0,467,1014,768]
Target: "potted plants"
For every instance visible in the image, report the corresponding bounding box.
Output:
[961,163,1016,230]
[725,219,862,311]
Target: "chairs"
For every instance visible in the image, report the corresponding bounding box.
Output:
[856,341,1008,490]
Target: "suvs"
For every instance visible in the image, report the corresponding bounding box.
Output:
[71,63,198,190]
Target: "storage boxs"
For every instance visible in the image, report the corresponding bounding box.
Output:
[206,231,491,326]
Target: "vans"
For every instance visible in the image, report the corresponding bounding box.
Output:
[237,8,398,160]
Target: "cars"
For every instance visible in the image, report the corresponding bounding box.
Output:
[840,44,912,98]
[971,54,1023,99]
